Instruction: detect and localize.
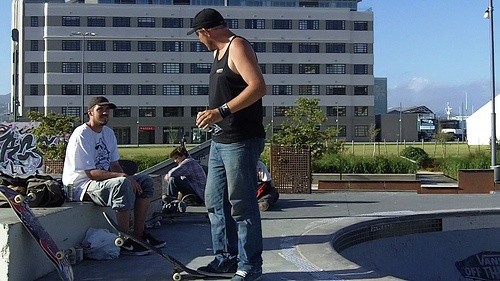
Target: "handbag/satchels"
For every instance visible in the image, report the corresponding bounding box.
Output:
[0,171,64,207]
[82,228,120,261]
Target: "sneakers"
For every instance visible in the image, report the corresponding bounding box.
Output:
[258,202,269,211]
[120,238,151,255]
[197,250,241,277]
[231,267,262,281]
[131,230,166,248]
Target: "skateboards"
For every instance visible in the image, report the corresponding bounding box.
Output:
[0,185,74,281]
[102,211,206,281]
[145,216,162,229]
[182,194,196,206]
[152,212,210,225]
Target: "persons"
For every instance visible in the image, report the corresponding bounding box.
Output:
[251,160,279,212]
[162,146,207,206]
[62,97,167,256]
[186,9,266,281]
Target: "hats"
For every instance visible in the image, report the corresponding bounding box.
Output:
[187,8,224,35]
[84,97,117,115]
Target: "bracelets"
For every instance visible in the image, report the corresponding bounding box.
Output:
[218,103,232,120]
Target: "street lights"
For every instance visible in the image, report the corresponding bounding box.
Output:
[72,31,95,124]
[483,6,496,165]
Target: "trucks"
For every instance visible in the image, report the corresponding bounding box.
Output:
[440,119,466,140]
